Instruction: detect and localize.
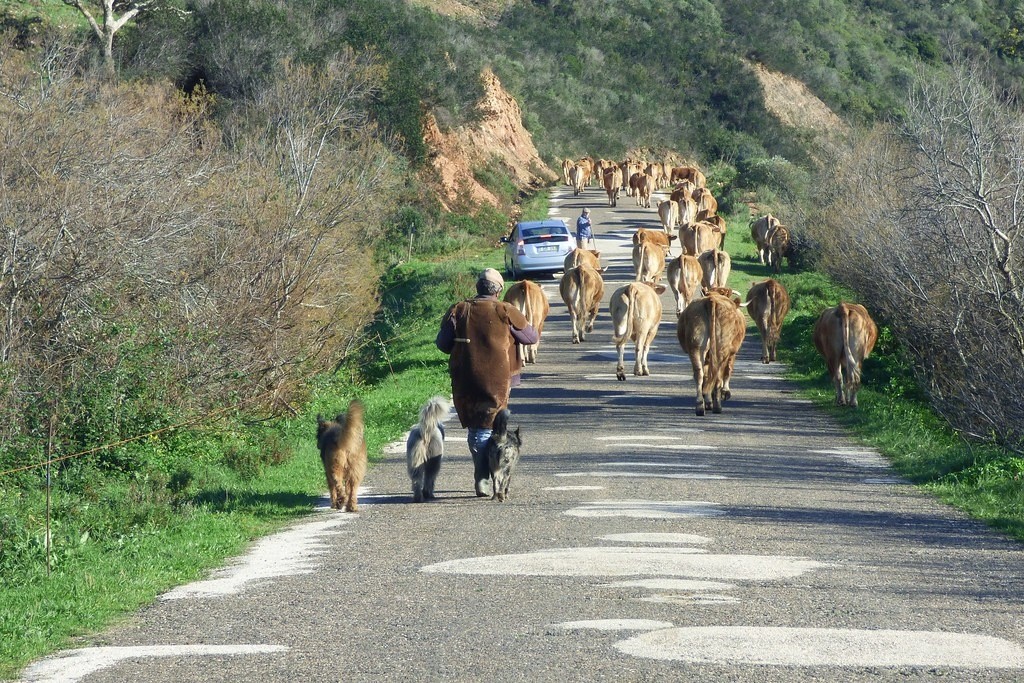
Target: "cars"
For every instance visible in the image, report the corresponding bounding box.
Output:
[500,220,578,280]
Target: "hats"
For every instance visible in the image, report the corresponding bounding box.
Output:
[480,267,505,291]
[583,208,590,213]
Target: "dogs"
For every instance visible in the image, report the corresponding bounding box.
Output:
[407,396,451,503]
[487,408,522,502]
[316,399,368,512]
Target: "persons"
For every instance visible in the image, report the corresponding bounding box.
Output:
[576,208,591,250]
[435,269,537,495]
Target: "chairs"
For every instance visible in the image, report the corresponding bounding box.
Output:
[549,228,563,234]
[522,231,535,237]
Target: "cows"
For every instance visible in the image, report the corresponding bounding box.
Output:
[677,288,758,416]
[667,254,704,319]
[503,280,549,367]
[697,249,731,293]
[811,302,878,408]
[746,277,790,365]
[559,265,610,344]
[632,242,670,281]
[561,157,726,258]
[749,212,781,266]
[609,281,669,381]
[764,225,792,273]
[564,247,601,272]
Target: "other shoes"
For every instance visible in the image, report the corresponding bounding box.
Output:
[476,488,489,497]
